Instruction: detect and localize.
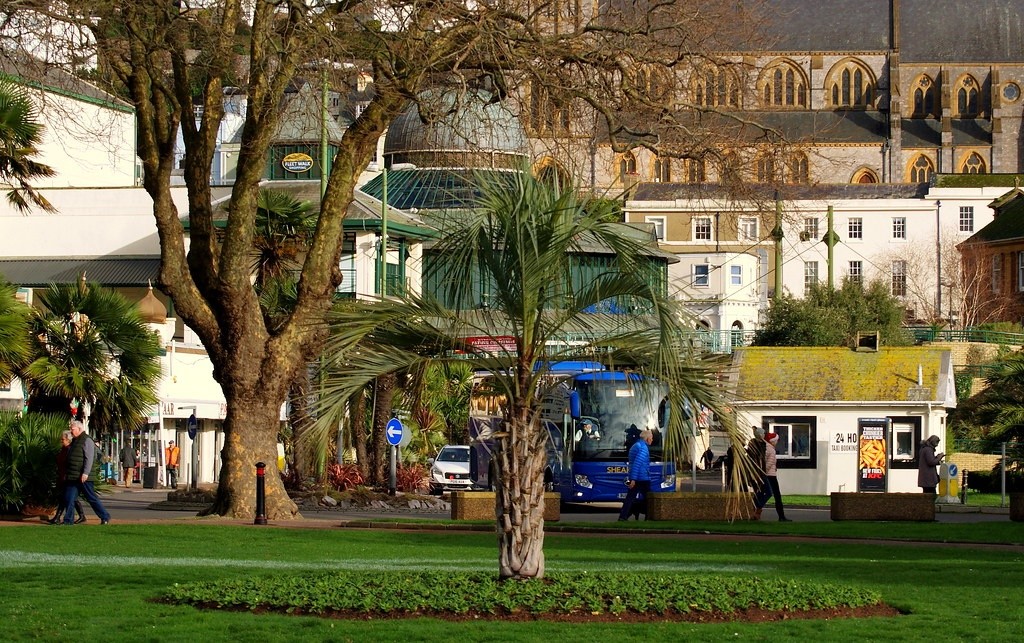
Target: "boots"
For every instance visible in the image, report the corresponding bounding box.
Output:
[49,503,66,524]
[74,501,87,524]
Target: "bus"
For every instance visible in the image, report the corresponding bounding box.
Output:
[469,361,676,512]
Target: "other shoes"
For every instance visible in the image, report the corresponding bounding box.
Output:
[778,517,793,522]
[100,514,111,525]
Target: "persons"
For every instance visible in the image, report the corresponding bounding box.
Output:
[575,419,600,442]
[918,435,945,522]
[119,443,137,488]
[726,434,749,492]
[700,446,713,468]
[47,431,86,525]
[93,440,110,482]
[764,433,793,522]
[165,440,180,489]
[747,426,772,520]
[60,421,111,525]
[617,430,654,521]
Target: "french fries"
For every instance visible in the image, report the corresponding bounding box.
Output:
[858,433,886,475]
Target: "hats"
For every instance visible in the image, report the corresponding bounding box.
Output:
[582,419,592,425]
[169,440,174,444]
[765,433,779,444]
[126,440,131,444]
[751,425,765,438]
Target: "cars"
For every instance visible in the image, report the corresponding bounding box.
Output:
[427,444,474,495]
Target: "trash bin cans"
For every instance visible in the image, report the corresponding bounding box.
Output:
[1009,492,1024,522]
[143,467,155,488]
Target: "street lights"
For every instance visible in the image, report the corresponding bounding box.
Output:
[364,162,417,300]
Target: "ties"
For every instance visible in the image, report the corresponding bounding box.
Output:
[587,432,589,435]
